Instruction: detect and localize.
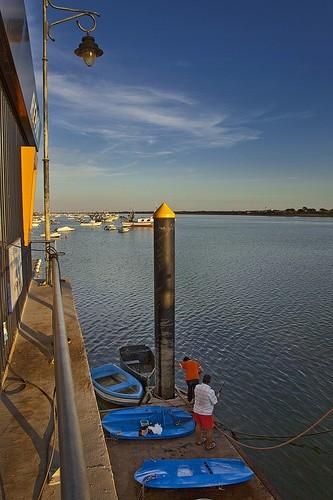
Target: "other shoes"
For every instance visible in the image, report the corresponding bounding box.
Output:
[205,442,216,450]
[196,437,207,445]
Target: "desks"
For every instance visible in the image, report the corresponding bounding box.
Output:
[124,359,140,373]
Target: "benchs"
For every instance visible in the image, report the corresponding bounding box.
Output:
[106,381,133,392]
[94,371,117,379]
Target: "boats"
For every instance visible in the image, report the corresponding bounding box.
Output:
[54,225,75,232]
[118,343,157,387]
[39,232,61,238]
[104,224,117,231]
[133,457,254,489]
[118,227,130,233]
[33,210,155,228]
[89,363,144,405]
[100,406,197,440]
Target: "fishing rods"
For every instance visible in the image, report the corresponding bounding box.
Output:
[217,356,245,394]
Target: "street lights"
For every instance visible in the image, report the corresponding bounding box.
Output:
[42,0,104,286]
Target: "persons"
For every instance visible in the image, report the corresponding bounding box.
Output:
[192,374,218,450]
[174,357,203,404]
